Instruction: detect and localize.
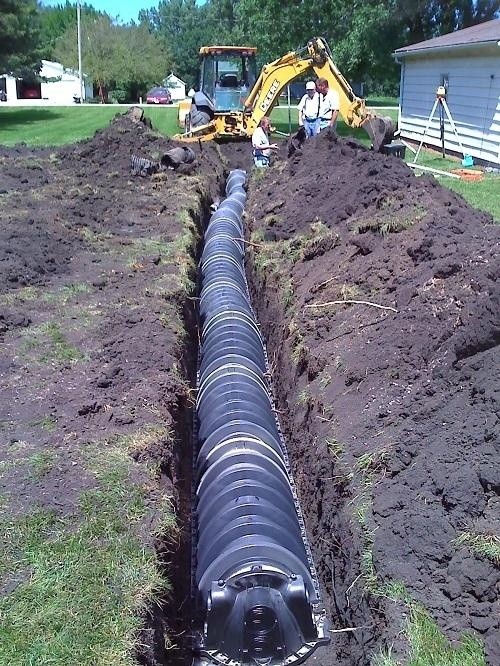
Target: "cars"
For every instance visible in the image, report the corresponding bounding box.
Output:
[146,88,171,104]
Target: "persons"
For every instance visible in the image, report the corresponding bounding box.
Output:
[251,116,279,168]
[297,78,340,139]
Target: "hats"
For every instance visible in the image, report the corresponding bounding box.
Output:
[305,80,315,91]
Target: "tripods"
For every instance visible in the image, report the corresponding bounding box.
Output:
[412,95,466,164]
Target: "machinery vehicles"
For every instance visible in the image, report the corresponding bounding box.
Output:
[170,36,394,153]
